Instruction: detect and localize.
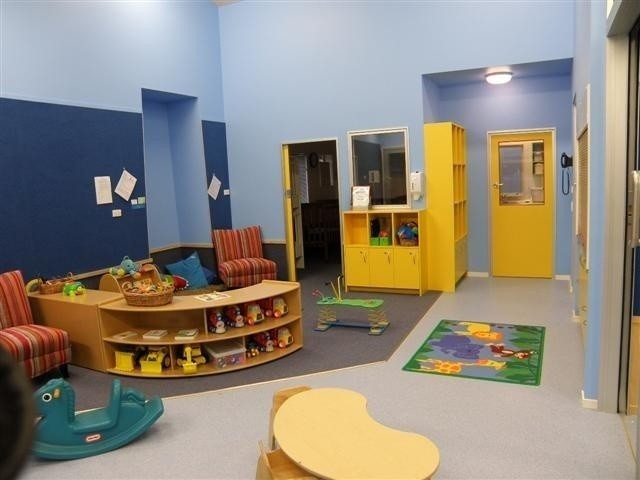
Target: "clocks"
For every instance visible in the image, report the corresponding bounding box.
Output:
[309,151,318,168]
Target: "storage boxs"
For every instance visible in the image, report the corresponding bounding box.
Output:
[204,341,249,371]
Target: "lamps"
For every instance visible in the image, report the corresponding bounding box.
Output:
[487,71,513,86]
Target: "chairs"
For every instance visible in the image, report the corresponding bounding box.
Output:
[300,197,340,262]
[255,440,318,480]
[266,384,312,450]
[0,270,73,381]
[212,224,278,286]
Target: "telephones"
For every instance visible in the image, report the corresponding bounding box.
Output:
[561,153,572,168]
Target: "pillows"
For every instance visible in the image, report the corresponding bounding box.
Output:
[166,251,215,290]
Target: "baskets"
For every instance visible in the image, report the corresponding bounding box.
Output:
[39,271,74,295]
[120,281,175,307]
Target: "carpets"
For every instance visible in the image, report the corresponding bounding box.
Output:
[401,319,547,387]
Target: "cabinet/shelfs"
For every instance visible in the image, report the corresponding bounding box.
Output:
[424,121,470,294]
[531,142,544,176]
[98,279,304,376]
[342,208,428,296]
[98,264,161,293]
[29,286,127,374]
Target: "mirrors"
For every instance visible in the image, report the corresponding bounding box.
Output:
[347,126,412,210]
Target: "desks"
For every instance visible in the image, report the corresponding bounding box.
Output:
[274,388,441,480]
